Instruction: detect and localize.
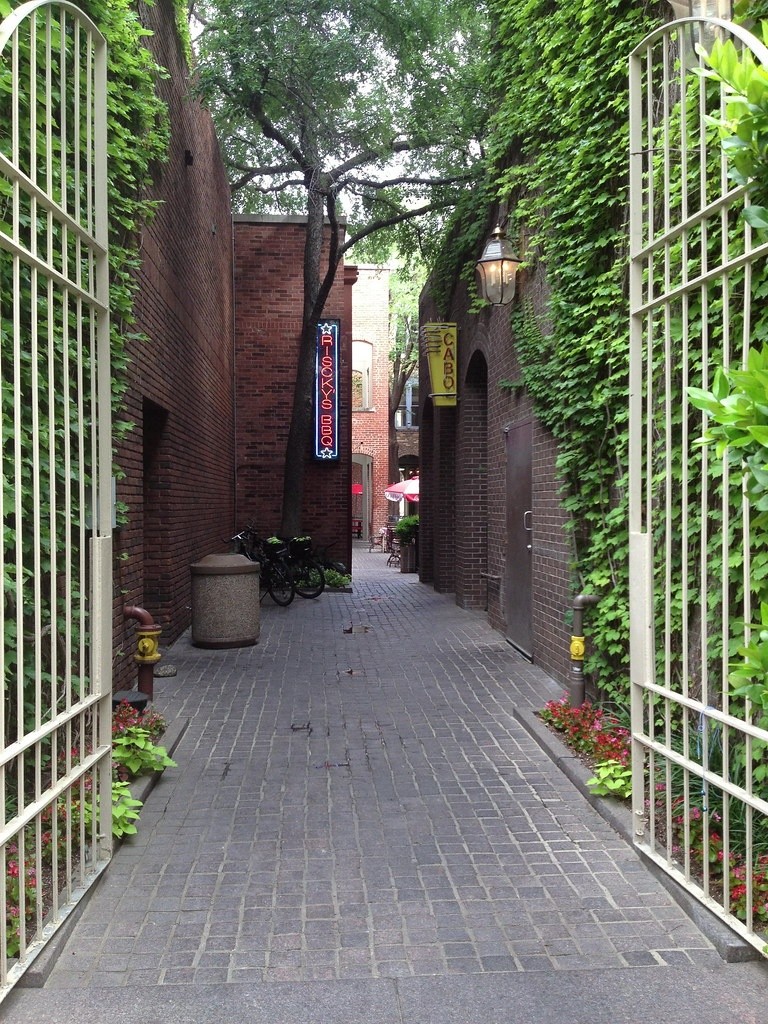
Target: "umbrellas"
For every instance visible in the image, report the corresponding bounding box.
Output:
[384,476,420,503]
[352,482,364,516]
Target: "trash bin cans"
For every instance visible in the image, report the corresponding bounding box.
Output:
[188,553,263,649]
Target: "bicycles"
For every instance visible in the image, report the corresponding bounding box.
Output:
[220,517,296,606]
[248,526,325,599]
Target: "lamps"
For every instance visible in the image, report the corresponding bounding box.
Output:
[473,215,537,308]
